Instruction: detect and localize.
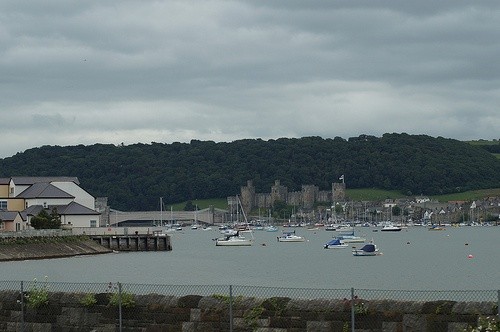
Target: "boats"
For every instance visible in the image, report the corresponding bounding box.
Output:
[276,229,305,243]
[351,236,385,258]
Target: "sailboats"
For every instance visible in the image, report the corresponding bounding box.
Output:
[215,195,256,246]
[151,194,493,250]
